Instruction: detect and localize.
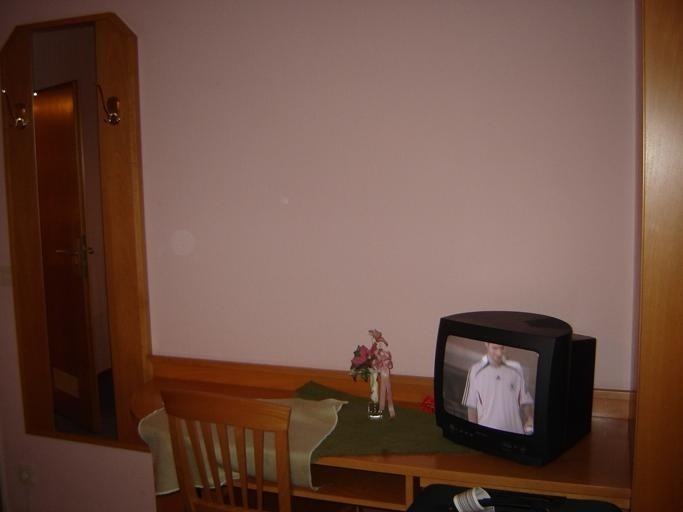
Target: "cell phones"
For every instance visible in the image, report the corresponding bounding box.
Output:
[367,400,383,419]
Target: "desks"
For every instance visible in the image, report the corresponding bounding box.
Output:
[130,377,632,511]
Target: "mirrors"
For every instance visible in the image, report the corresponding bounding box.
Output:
[29,22,119,440]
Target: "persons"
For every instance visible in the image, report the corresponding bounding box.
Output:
[460,342,533,437]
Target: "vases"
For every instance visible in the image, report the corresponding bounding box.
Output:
[368,373,383,418]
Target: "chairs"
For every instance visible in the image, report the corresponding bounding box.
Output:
[159,387,292,511]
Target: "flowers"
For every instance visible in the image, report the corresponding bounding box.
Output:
[348,330,393,413]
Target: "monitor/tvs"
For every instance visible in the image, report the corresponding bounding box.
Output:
[432,310,597,466]
